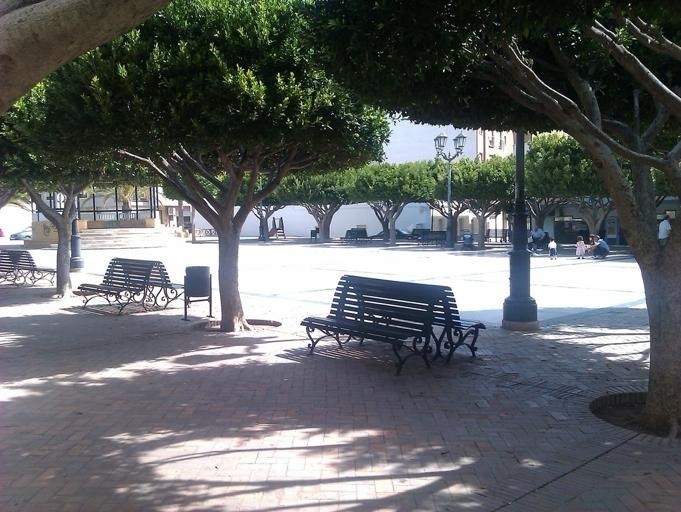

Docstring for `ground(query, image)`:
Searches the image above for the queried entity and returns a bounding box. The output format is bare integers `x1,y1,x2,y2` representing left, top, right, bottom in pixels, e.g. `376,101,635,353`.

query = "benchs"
77,257,184,316
299,275,487,377
411,228,447,248
0,249,58,287
340,228,373,244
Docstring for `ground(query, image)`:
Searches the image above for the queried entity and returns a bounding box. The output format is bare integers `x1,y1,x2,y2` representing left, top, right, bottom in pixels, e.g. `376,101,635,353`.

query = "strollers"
462,231,473,250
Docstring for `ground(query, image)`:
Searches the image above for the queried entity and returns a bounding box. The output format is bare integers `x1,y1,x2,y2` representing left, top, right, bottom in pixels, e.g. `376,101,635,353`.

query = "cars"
368,227,420,239
9,226,30,242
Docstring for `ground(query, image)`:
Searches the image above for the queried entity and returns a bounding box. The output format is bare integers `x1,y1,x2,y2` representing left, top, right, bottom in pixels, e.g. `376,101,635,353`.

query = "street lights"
434,129,468,247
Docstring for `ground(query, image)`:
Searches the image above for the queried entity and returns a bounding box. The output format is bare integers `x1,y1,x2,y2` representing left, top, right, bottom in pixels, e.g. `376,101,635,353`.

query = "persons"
588,236,610,259
548,238,558,261
575,236,586,259
659,214,672,249
530,225,546,250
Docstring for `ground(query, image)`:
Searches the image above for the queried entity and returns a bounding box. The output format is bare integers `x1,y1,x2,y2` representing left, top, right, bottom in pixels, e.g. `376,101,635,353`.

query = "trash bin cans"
181,266,214,320
311,230,317,240
463,233,473,245
